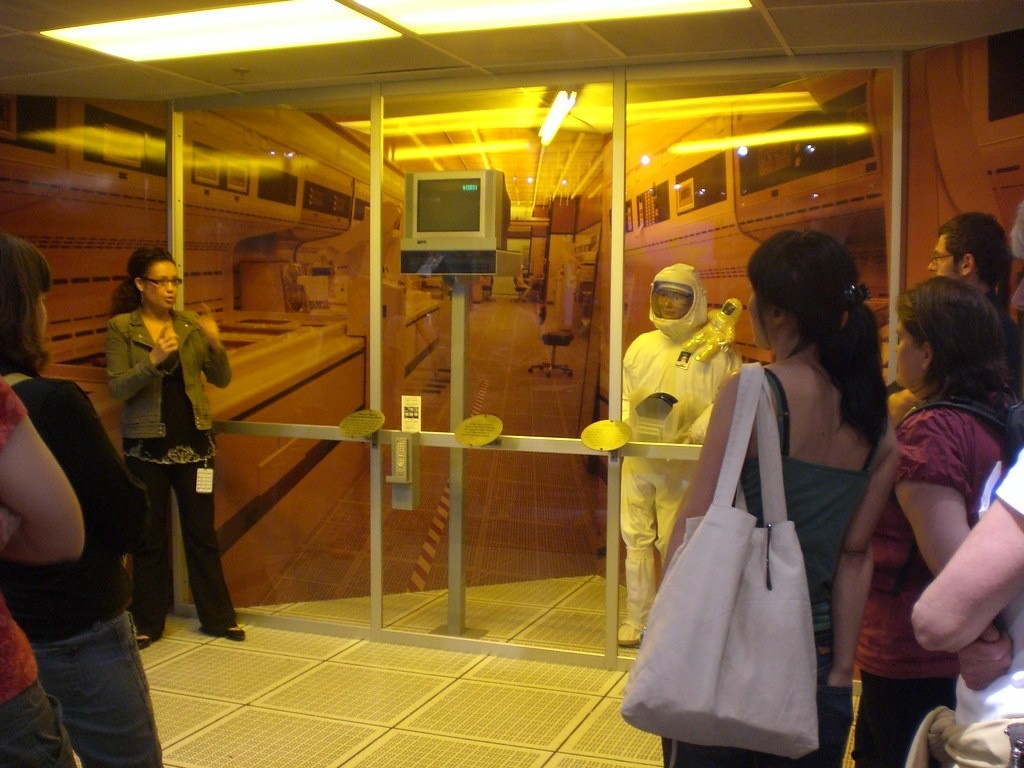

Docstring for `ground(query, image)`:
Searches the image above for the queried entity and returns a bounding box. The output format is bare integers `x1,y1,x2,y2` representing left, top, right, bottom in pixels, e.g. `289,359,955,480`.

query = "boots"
618,546,656,646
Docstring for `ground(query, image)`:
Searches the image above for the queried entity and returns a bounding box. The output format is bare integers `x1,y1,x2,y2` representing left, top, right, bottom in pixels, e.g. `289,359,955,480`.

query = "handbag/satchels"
621,359,820,758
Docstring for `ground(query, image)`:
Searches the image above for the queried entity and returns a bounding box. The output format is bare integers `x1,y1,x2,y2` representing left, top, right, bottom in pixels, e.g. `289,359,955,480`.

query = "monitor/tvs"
399,168,511,251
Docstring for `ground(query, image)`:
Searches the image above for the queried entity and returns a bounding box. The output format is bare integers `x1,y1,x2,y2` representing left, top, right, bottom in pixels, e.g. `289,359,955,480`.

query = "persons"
683,298,743,363
661,228,896,768
912,201,1024,768
0,234,162,768
928,212,1020,389
106,245,245,648
0,377,86,767
514,265,531,301
617,264,744,645
851,278,1007,767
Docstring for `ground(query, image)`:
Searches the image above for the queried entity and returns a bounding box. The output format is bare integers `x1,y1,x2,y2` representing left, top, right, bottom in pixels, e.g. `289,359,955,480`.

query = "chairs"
529,301,574,378
482,276,496,302
510,276,527,303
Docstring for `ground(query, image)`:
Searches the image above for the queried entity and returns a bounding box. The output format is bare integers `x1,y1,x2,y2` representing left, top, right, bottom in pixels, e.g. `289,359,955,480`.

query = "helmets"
648,264,709,338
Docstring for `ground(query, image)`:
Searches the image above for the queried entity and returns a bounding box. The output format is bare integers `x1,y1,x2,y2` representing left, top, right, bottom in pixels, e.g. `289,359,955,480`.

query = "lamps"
537,88,580,147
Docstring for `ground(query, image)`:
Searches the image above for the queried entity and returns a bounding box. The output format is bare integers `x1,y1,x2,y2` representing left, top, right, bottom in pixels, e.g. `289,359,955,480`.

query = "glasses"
929,253,954,265
141,275,182,290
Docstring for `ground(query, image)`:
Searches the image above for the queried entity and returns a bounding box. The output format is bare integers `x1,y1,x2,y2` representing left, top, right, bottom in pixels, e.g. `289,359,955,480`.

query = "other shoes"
199,624,245,641
135,632,163,650
